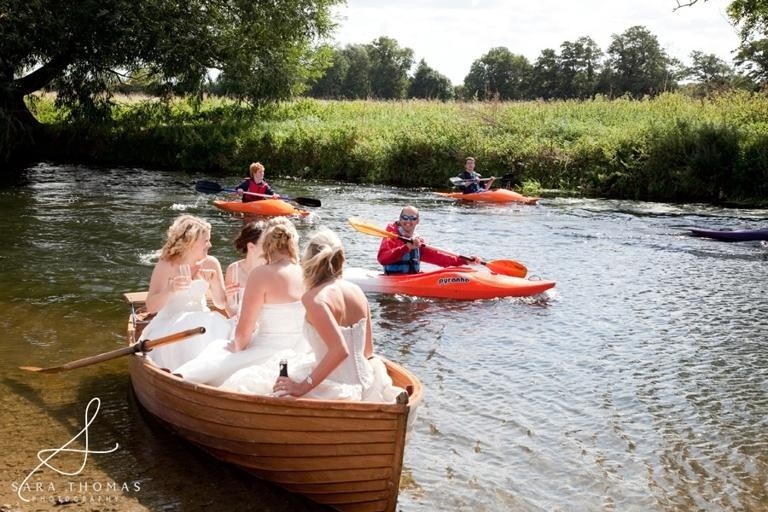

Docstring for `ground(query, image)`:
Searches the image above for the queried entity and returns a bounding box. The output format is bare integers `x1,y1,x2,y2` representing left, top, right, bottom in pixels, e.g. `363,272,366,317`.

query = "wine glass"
179,265,198,308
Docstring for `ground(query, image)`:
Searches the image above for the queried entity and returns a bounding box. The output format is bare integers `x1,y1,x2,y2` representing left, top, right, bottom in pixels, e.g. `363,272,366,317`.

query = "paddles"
348,217,527,278
449,173,513,186
18,327,206,372
195,180,321,208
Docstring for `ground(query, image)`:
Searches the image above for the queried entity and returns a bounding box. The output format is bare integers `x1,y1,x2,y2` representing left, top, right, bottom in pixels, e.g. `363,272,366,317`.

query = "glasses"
401,215,418,220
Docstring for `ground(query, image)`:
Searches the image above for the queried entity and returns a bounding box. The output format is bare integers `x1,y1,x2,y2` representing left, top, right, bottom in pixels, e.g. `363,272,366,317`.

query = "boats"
124,289,420,510
447,188,538,206
344,258,558,302
212,199,315,219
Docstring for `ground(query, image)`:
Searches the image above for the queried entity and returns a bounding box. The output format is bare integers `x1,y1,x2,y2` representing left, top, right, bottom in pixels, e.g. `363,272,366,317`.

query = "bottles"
274,359,291,398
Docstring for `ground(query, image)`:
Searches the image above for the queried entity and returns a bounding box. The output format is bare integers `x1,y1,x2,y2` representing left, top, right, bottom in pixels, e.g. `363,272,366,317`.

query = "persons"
135,162,405,404
377,206,482,275
453,157,496,193
